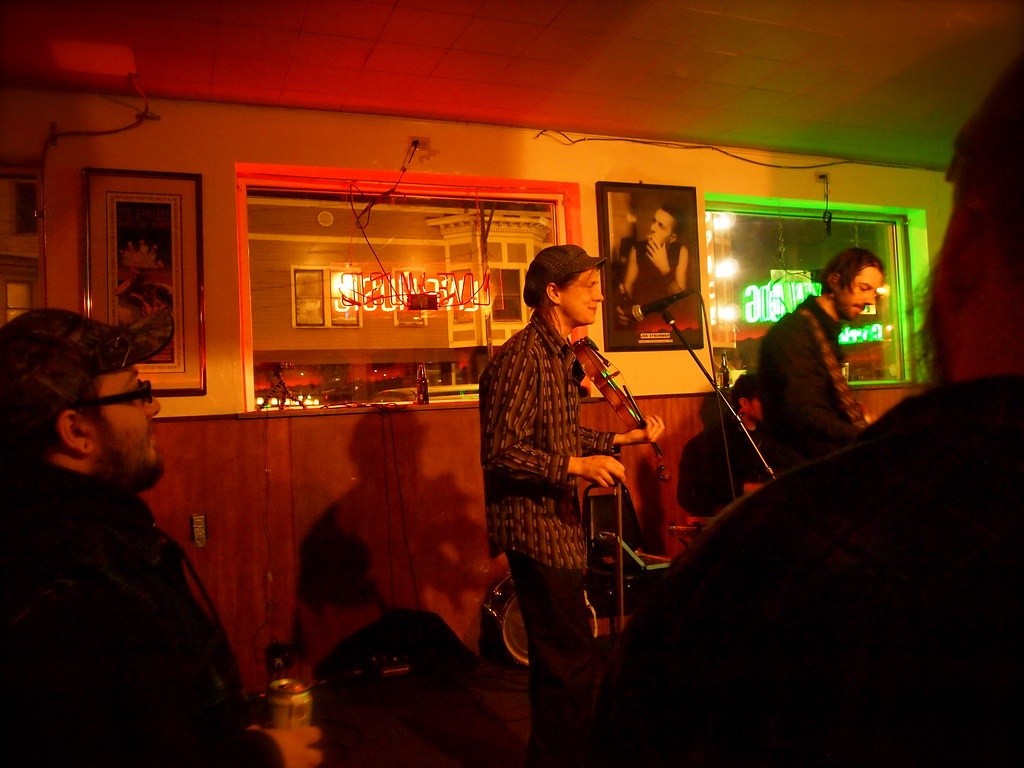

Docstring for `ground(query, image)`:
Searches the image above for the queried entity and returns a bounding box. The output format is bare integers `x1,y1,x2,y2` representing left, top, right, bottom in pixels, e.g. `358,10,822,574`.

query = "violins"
570,336,674,484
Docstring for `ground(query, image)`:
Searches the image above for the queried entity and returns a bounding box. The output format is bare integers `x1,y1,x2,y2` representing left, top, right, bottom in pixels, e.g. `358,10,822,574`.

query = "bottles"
617,282,638,327
415,362,430,405
717,353,729,389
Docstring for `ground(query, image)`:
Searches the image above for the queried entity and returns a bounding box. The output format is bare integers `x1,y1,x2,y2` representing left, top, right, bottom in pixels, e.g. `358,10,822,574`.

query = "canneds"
262,678,312,727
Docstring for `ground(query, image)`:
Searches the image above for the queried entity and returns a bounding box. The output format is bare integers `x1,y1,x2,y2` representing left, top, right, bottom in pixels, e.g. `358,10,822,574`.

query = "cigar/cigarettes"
645,234,653,238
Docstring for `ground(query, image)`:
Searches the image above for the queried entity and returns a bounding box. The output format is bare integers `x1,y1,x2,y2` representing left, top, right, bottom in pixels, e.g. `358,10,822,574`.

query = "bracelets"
663,270,675,283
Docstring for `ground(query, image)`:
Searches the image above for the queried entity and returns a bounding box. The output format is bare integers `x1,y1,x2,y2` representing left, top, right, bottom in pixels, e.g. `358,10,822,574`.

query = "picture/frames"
80,166,209,398
392,266,428,328
290,266,330,330
596,180,706,353
329,267,365,330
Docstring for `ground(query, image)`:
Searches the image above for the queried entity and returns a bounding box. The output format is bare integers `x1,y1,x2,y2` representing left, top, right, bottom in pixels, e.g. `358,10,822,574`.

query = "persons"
584,58,1024,768
614,205,689,328
477,244,666,768
0,309,325,768
712,374,791,514
759,249,887,468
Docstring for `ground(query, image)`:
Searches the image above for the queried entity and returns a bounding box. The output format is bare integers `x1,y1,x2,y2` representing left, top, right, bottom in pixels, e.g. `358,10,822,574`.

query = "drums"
485,566,598,670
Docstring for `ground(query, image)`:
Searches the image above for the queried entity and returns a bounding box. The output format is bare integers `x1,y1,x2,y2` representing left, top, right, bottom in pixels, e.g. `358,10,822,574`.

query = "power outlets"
815,171,830,184
409,135,432,152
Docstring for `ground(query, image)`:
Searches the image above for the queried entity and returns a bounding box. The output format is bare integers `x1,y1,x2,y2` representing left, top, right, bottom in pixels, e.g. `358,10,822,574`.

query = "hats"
0,308,176,438
523,244,608,307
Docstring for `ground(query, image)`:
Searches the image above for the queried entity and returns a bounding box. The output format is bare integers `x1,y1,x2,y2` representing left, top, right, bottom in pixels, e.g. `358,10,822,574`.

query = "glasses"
66,378,152,409
